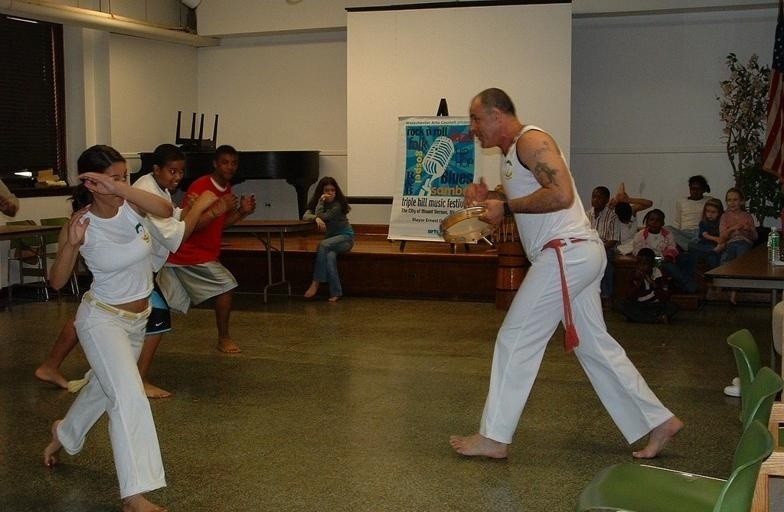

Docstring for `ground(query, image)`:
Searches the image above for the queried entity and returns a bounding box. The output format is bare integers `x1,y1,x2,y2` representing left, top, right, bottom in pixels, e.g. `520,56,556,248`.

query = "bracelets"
238,207,243,217
503,203,511,218
210,207,214,215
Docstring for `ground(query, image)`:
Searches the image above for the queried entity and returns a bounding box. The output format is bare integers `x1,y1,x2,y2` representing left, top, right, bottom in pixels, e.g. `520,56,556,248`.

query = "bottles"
768,226,780,264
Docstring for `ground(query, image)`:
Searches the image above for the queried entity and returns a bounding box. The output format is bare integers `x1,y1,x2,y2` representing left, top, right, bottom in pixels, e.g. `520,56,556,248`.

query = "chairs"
726,299,784,512
577,420,777,511
0,217,82,302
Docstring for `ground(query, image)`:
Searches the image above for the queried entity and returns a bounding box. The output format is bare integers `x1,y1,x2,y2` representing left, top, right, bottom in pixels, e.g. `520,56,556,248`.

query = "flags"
761,0,783,188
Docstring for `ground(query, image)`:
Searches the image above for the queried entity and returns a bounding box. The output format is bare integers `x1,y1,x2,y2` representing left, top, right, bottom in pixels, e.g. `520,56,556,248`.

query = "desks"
702,238,784,398
222,217,314,304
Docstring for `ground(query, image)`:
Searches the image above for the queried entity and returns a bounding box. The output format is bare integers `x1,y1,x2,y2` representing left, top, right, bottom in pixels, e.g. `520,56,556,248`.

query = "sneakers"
724,376,741,396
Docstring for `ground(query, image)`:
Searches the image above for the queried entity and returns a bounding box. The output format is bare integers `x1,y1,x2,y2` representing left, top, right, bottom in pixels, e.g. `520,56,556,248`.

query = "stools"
172,109,219,151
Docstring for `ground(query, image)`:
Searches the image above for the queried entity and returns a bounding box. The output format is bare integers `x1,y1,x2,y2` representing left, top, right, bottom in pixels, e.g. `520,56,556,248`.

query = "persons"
584,174,758,323
303,176,354,304
0,179,19,217
34,143,218,399
450,88,683,459
158,144,255,352
44,144,174,511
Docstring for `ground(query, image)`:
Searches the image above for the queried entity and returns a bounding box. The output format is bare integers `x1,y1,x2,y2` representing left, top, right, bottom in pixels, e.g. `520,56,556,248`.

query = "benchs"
600,254,672,312
